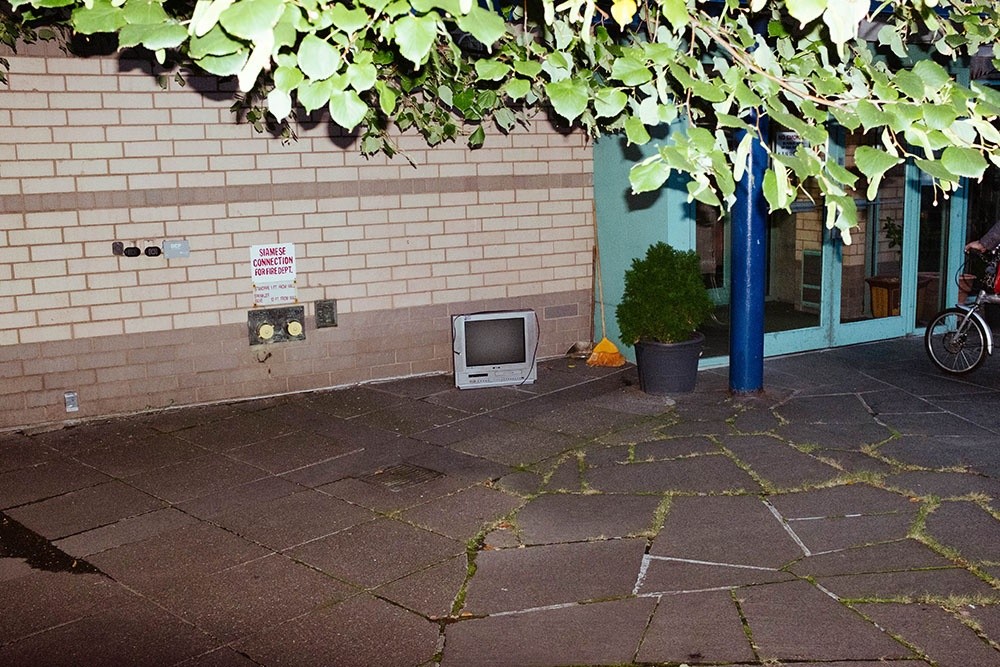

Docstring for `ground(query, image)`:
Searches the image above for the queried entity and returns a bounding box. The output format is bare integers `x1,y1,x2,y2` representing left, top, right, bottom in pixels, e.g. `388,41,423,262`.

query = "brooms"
584,199,626,367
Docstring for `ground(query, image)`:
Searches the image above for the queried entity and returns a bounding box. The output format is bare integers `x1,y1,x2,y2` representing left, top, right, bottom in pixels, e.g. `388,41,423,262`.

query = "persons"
965,221,1000,254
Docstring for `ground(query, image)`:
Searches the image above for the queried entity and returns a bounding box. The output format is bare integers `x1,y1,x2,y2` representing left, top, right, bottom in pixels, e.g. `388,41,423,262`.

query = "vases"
922,271,976,322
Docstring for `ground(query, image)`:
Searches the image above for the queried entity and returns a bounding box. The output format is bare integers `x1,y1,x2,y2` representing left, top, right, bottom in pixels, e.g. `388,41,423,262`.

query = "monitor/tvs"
451,309,537,390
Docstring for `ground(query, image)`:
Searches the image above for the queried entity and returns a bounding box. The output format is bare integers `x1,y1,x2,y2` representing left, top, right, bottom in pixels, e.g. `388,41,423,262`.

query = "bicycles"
924,240,1000,376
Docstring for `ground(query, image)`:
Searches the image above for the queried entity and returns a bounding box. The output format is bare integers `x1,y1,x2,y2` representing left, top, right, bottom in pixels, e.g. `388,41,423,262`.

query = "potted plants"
614,240,717,395
864,216,931,327
